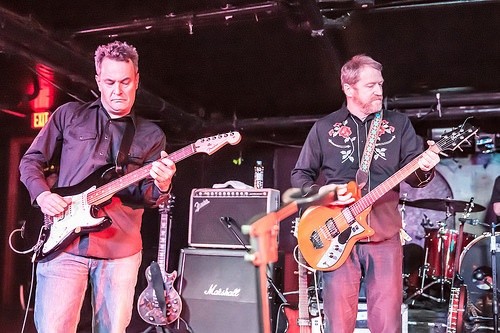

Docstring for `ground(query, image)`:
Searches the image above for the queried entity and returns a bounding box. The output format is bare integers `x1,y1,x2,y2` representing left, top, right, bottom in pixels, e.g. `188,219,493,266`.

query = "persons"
19,42,176,333
291,55,440,333
486,175,500,231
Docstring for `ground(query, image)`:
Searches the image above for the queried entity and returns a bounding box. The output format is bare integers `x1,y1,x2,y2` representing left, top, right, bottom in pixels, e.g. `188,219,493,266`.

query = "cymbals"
399,197,416,207
415,198,486,213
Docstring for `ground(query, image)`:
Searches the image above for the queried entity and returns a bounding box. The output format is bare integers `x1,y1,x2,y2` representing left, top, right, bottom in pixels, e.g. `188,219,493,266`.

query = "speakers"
168,249,279,333
189,187,281,250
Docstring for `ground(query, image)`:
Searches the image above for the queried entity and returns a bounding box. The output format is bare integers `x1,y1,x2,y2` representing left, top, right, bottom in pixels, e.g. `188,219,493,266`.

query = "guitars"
274,217,323,333
136,194,184,326
296,115,479,272
23,130,243,263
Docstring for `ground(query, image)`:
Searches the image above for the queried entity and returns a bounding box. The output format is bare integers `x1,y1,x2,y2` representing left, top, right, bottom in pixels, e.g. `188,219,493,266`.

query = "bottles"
253,161,264,189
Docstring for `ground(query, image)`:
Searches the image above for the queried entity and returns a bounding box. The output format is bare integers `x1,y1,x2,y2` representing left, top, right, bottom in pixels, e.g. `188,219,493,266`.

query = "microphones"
221,216,233,222
436,93,442,117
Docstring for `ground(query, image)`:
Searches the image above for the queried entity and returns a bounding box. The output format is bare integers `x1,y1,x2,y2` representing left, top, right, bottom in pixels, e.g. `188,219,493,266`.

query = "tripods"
403,204,453,307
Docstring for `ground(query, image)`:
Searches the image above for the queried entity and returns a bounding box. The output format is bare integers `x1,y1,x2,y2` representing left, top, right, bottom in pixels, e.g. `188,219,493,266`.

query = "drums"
424,227,477,282
402,243,428,305
459,231,500,318
